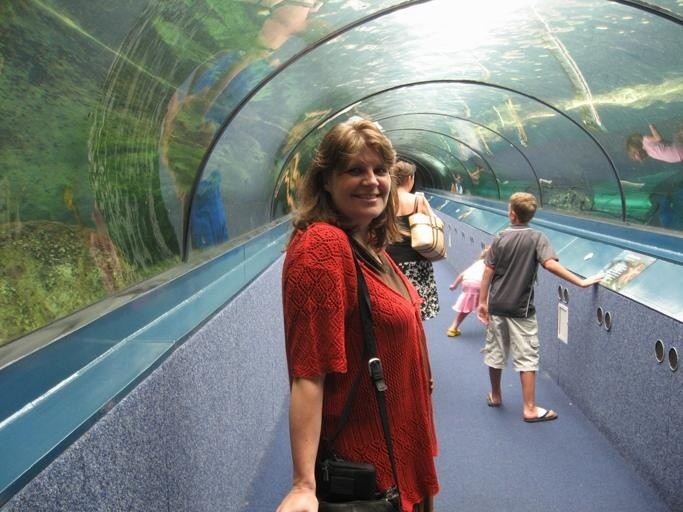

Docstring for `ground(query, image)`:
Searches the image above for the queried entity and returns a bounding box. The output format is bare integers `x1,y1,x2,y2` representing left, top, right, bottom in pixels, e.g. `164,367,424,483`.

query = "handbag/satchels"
409,213,448,262
314,457,402,512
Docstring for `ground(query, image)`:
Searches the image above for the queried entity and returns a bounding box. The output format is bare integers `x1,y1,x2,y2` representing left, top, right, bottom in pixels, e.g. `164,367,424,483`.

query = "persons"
475,191,607,423
273,114,441,512
389,160,440,321
626,124,683,164
446,243,493,337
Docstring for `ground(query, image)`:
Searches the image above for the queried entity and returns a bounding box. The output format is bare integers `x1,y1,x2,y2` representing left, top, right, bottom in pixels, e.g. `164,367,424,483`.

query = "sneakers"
447,329,461,337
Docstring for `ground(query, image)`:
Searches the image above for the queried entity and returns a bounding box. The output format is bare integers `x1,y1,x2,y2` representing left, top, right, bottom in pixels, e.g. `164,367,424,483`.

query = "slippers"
487,393,497,406
524,407,557,422
